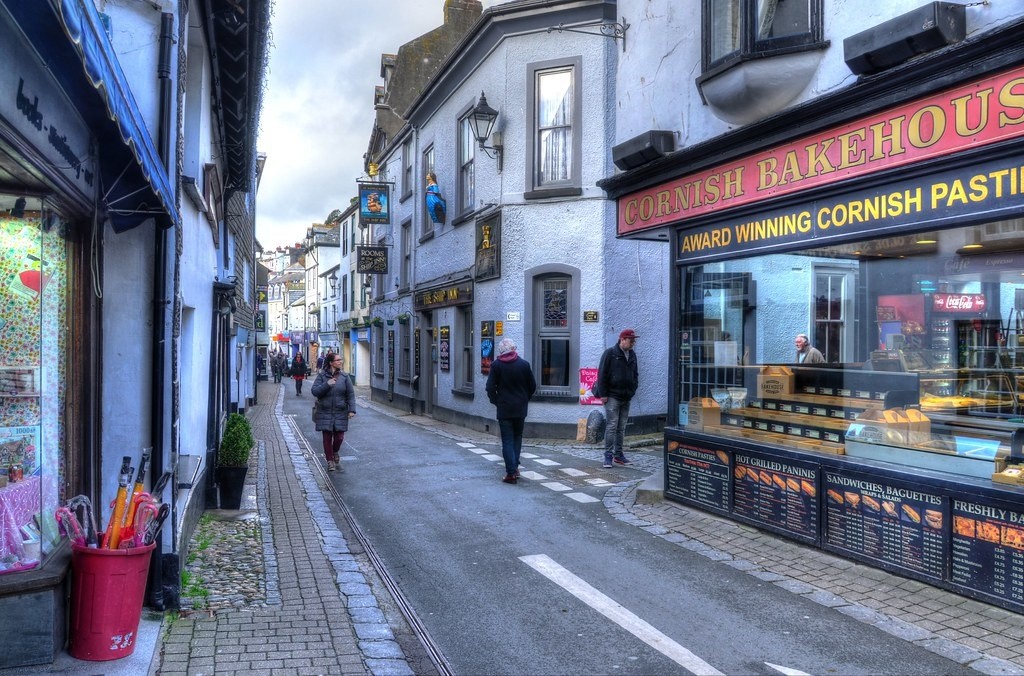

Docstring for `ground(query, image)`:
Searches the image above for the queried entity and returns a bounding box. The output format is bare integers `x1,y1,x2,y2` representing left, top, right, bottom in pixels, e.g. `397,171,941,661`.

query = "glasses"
297,354,300,355
333,359,342,362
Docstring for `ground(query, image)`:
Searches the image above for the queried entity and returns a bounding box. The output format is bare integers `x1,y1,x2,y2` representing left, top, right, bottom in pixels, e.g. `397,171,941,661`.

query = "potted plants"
373,316,382,327
399,314,407,324
215,412,256,509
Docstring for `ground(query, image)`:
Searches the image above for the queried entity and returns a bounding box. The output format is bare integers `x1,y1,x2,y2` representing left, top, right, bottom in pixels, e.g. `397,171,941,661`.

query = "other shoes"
327,452,339,471
503,469,521,484
296,391,302,396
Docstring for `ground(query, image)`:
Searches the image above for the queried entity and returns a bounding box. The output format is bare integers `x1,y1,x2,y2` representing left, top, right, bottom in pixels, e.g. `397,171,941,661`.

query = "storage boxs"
0,366,40,398
0,425,40,477
688,366,930,455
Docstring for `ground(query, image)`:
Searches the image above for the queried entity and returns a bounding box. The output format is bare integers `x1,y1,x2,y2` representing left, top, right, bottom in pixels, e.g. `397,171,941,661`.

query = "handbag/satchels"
312,401,318,423
592,381,600,398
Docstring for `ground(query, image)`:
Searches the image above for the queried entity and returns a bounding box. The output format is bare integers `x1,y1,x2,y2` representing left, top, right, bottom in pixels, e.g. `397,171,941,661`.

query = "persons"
311,352,356,471
425,173,446,223
268,351,288,383
597,329,641,469
793,334,826,364
485,338,537,485
315,349,333,374
290,351,308,396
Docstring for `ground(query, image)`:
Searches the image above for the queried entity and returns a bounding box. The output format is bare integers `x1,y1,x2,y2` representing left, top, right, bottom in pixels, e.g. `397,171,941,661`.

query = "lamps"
703,289,713,297
10,195,26,218
36,208,58,235
328,270,341,289
403,310,419,319
467,90,503,176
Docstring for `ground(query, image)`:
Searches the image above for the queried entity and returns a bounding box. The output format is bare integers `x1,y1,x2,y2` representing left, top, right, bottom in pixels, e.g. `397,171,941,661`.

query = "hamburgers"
734,466,746,479
924,510,942,530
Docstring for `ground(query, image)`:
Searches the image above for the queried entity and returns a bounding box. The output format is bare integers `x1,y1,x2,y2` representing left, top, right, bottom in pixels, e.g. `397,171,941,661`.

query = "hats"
620,329,640,339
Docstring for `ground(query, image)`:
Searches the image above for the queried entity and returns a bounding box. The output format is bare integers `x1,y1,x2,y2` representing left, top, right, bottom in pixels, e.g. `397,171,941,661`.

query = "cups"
23,539,41,563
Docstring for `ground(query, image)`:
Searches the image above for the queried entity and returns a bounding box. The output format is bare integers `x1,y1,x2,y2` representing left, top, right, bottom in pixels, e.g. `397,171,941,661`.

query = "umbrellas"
55,454,170,551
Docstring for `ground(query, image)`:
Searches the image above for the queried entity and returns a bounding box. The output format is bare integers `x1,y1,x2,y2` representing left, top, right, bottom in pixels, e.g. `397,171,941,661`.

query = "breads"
960,401,977,406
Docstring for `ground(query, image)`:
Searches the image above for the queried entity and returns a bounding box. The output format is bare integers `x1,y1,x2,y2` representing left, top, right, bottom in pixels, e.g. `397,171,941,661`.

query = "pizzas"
957,517,1024,550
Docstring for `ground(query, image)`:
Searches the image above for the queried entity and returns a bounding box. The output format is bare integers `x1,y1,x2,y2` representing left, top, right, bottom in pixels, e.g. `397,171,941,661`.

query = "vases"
387,320,394,325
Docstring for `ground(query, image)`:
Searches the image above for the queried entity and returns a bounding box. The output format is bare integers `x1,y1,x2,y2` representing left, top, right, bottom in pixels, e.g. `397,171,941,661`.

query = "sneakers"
614,456,633,466
603,456,613,468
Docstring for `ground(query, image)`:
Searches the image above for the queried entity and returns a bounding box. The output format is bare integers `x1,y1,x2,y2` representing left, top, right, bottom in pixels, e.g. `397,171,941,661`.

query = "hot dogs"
746,466,921,524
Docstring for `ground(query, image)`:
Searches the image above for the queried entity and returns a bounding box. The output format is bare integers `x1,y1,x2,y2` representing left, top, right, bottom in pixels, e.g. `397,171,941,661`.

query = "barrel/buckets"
67,533,157,660
